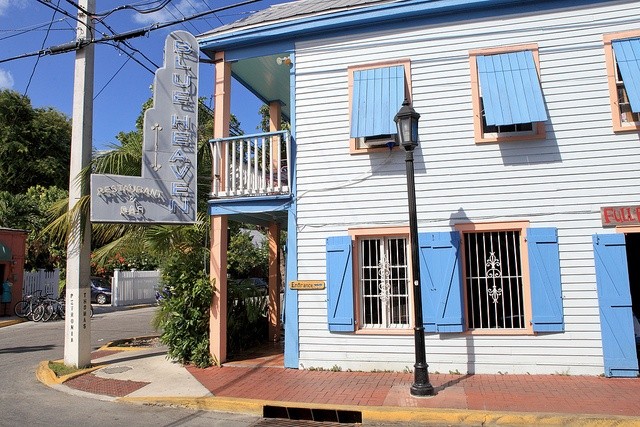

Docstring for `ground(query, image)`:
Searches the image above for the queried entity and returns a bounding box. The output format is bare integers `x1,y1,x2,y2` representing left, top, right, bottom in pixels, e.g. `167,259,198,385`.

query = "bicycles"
13,287,65,323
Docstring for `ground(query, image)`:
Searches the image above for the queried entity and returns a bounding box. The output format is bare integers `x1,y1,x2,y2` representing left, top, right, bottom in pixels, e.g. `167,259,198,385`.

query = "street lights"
393,97,435,395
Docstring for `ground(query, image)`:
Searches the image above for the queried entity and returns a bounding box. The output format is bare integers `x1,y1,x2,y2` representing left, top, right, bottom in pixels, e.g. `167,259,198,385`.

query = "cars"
91,276,111,303
